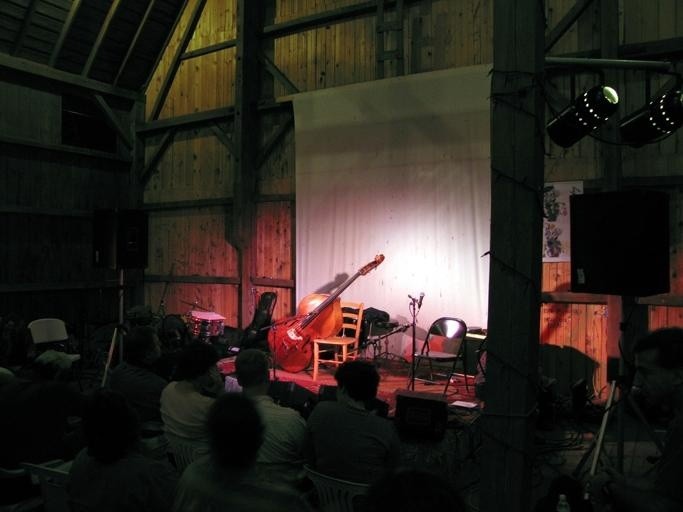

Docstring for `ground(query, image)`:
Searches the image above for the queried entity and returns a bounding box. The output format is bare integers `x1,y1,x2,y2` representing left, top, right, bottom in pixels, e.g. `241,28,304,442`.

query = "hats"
177,340,226,377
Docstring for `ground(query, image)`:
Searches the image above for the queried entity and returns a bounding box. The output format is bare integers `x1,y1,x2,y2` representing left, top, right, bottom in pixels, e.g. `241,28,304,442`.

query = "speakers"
570,189,670,297
91,209,148,270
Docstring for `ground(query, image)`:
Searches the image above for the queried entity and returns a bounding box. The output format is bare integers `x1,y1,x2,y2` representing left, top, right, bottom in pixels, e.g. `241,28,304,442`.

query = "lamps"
545,71,683,149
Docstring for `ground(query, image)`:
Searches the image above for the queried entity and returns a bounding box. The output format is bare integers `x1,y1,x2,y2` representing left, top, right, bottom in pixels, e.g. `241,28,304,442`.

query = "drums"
184,311,226,343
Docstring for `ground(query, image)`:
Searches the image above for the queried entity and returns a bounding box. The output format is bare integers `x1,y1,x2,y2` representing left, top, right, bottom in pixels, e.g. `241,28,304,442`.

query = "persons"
631,326,682,510
0,306,412,512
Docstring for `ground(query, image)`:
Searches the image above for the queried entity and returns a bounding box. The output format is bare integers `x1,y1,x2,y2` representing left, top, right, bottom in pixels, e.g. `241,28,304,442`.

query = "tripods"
101,270,126,388
573,295,663,485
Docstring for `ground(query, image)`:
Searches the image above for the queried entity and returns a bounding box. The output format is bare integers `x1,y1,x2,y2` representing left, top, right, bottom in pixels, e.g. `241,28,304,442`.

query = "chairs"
1,458,80,512
305,463,370,512
310,300,363,379
26,318,86,392
158,407,209,483
403,316,472,398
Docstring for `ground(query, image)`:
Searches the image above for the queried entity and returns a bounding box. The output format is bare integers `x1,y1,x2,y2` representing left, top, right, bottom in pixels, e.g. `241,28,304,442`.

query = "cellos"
265,253,388,372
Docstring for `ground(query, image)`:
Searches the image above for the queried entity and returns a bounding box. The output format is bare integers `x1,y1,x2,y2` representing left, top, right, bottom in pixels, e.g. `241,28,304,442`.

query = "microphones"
418,292,425,308
